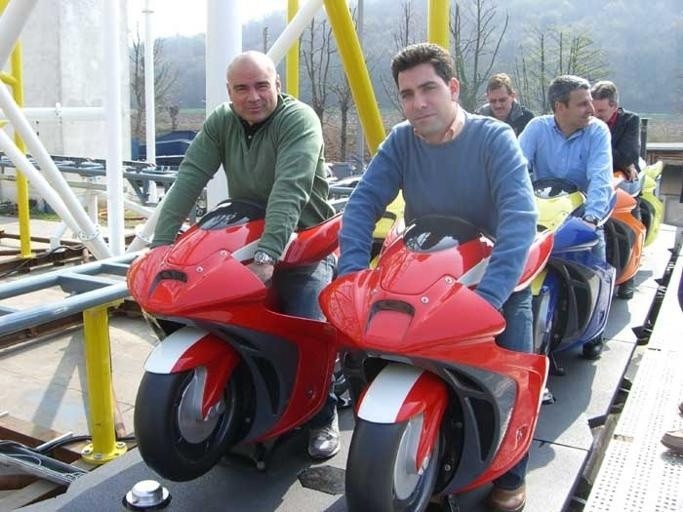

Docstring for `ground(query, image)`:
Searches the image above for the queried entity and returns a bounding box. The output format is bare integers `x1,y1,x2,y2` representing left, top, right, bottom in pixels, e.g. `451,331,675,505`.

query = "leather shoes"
490,449,530,512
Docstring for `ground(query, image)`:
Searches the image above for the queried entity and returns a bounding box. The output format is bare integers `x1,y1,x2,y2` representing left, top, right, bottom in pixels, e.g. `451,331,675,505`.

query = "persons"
516,73,615,358
661,268,683,454
589,78,641,298
149,50,344,462
335,40,540,512
472,71,536,140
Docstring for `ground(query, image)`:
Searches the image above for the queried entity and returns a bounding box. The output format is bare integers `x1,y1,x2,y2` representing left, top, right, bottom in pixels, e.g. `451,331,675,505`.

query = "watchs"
582,214,600,228
252,251,275,266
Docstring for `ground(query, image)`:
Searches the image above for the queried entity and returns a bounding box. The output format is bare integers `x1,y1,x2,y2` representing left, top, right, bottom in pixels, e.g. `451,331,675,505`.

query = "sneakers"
582,333,602,358
618,277,634,301
308,407,339,461
